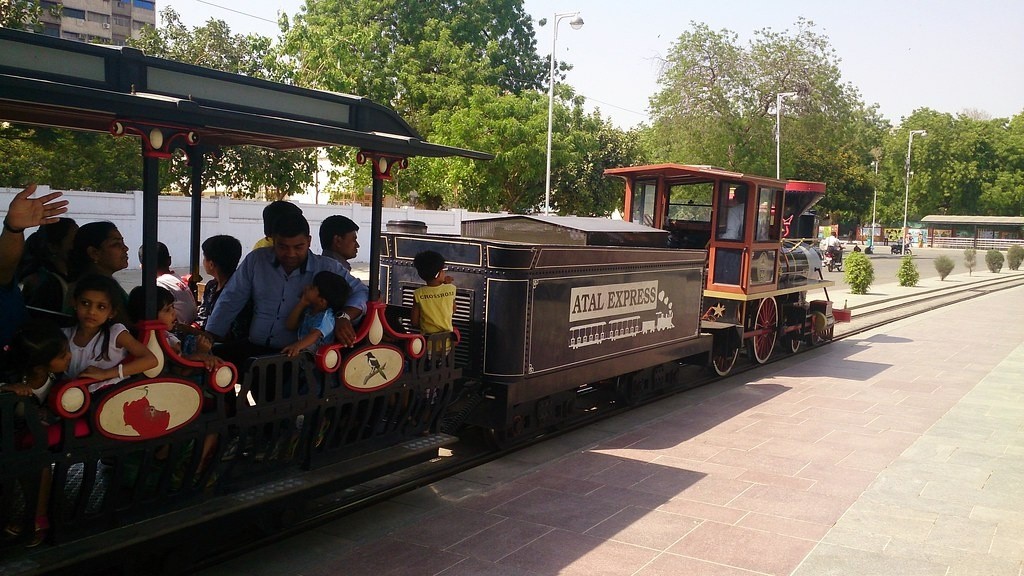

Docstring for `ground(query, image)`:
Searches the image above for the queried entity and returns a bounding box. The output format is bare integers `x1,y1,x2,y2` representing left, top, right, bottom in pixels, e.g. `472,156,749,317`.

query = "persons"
884,230,890,246
0,183,458,540
705,184,746,266
918,234,923,248
827,232,845,266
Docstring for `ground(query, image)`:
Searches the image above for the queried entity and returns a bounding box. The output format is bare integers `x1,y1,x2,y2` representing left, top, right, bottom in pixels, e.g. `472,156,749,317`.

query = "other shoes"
176,418,332,491
24,522,54,547
3,517,28,537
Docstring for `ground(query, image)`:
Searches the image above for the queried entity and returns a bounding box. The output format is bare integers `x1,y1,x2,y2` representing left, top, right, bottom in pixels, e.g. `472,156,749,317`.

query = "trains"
0,22,852,576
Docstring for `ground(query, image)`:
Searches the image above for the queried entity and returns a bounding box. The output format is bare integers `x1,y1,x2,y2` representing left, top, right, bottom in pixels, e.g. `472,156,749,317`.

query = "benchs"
0,304,457,551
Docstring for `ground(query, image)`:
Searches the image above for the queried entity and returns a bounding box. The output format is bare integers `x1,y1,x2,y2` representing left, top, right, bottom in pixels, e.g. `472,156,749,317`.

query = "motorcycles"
825,247,846,272
891,241,912,255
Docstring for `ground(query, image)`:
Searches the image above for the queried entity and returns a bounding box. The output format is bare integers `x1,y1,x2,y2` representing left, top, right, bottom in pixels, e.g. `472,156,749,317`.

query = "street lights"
775,91,812,181
901,129,928,255
870,160,879,251
544,10,584,216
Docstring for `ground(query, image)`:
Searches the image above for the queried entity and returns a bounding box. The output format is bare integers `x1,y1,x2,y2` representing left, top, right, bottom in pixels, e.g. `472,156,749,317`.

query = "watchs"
335,310,351,321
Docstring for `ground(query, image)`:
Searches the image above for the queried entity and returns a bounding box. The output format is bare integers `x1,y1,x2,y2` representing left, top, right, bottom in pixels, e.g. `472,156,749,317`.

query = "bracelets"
192,330,195,333
118,363,124,378
4,216,25,233
